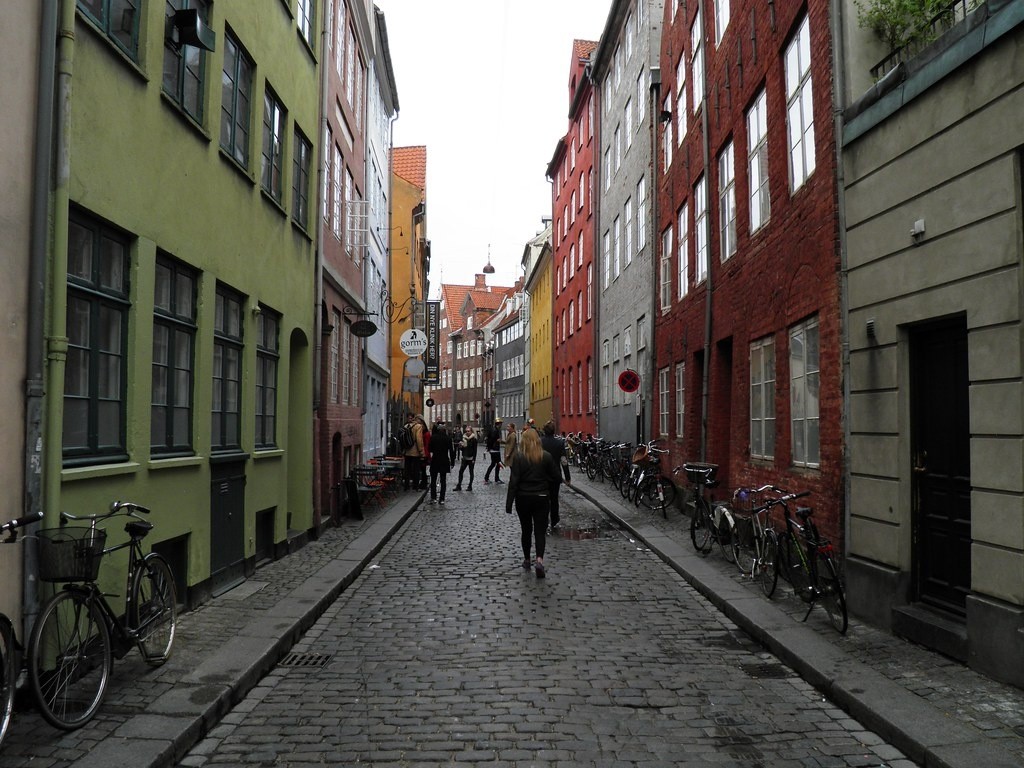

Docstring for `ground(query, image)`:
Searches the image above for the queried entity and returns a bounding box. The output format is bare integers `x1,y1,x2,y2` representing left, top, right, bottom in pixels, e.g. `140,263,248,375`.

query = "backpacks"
397,423,417,455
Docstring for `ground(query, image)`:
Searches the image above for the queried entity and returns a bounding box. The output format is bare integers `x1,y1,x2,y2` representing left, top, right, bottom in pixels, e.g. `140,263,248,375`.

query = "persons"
541,422,571,528
505,429,561,578
504,423,518,467
453,425,463,460
405,413,442,488
404,414,425,492
484,419,505,483
453,426,477,491
428,424,456,505
562,431,604,461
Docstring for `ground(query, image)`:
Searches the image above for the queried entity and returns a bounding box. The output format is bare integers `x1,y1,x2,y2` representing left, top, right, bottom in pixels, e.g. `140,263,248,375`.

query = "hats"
495,418,503,423
415,414,424,420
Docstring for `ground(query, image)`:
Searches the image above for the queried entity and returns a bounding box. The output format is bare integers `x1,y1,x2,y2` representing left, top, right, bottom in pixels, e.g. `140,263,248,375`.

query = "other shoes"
466,485,472,491
522,560,531,570
495,480,504,484
429,499,437,503
551,517,560,527
534,562,545,578
484,478,492,483
438,500,444,504
453,484,462,491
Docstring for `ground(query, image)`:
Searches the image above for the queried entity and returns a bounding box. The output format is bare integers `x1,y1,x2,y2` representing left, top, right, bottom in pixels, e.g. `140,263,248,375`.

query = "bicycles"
751,488,848,635
556,431,678,519
731,485,786,600
672,461,741,565
0,511,46,754
29,500,178,732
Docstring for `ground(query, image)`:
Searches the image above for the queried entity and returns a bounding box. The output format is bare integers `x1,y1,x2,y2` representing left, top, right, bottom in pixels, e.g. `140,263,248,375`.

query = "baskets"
32,526,107,584
632,446,651,468
686,461,719,486
